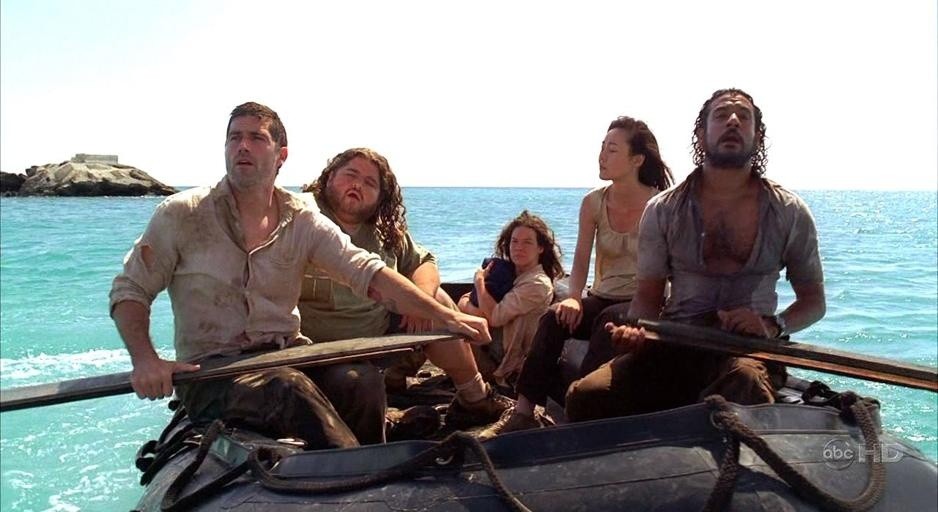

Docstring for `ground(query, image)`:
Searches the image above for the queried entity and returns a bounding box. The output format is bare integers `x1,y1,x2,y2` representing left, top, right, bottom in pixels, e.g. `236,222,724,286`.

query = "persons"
295,146,519,441
564,86,827,418
476,114,676,439
459,209,565,397
105,101,494,448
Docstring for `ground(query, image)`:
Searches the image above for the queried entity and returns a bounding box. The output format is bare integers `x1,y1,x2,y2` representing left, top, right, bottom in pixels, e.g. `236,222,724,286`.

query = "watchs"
762,312,788,339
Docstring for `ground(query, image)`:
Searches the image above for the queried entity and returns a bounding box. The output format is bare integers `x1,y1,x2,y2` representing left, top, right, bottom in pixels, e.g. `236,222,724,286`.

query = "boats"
134,282,938,511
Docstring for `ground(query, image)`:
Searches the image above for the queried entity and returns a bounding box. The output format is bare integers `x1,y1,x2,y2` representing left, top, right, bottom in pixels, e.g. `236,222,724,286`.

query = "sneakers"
446,382,517,430
477,407,541,440
